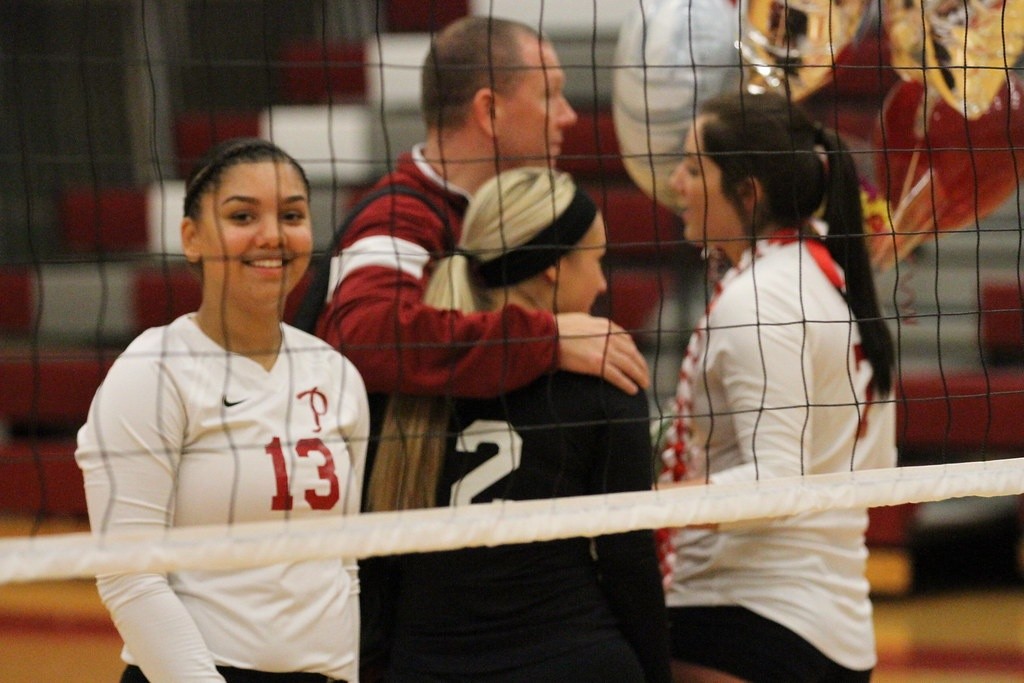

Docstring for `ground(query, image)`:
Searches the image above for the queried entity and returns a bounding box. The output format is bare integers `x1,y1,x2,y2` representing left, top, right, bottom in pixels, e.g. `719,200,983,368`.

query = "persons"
75,139,369,683
646,93,894,683
289,17,669,683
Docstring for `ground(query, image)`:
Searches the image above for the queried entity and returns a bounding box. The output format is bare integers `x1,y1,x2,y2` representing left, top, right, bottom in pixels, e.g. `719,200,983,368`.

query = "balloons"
612,0,1024,273
